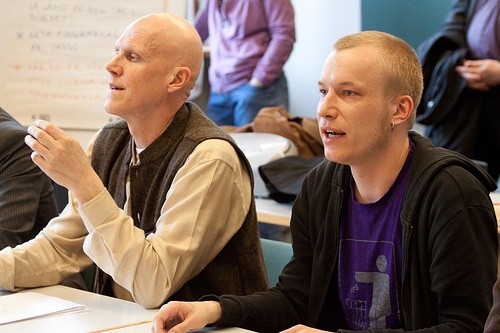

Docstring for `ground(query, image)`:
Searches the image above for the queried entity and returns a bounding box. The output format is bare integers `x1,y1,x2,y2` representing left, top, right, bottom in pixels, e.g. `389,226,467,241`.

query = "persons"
485,279,500,333
191,0,296,130
416,0,500,182
0,107,88,293
150,30,498,333
0,13,271,309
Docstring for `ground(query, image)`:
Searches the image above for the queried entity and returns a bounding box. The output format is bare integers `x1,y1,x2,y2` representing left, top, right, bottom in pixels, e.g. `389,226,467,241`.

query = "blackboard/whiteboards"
0,1,187,132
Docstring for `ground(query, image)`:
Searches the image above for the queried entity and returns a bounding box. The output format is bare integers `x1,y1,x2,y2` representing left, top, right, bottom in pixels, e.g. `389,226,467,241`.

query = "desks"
252,197,294,244
0,272,276,333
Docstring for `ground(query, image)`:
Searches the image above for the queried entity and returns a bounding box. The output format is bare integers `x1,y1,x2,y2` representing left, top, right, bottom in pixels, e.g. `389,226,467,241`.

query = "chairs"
258,238,295,287
